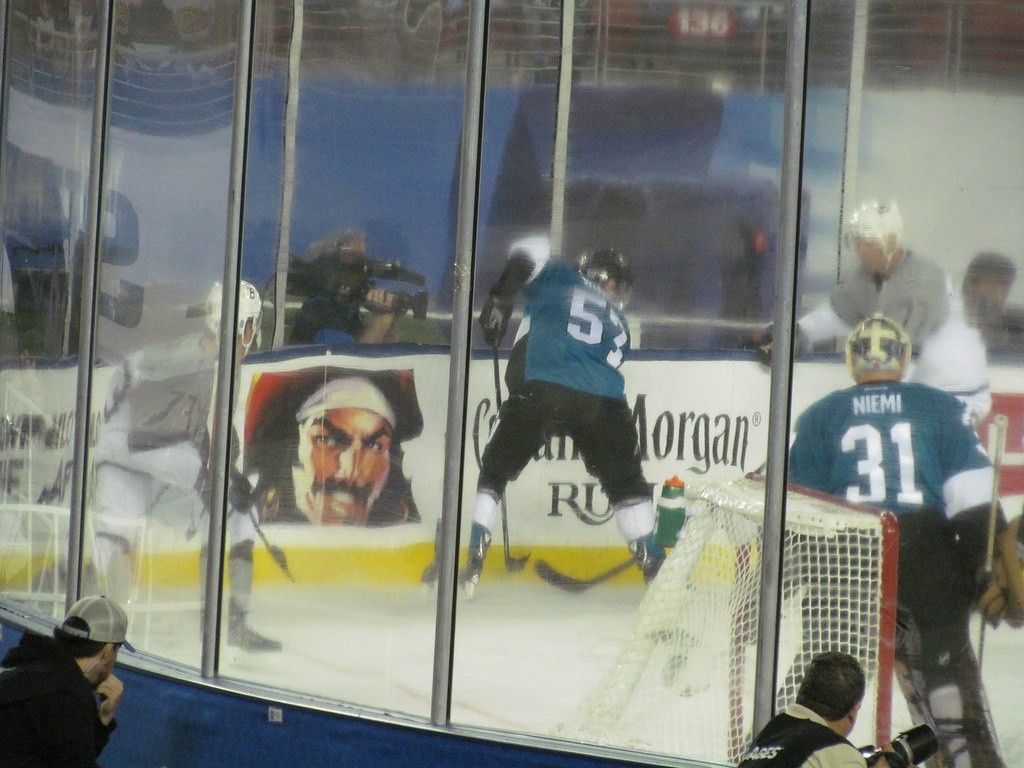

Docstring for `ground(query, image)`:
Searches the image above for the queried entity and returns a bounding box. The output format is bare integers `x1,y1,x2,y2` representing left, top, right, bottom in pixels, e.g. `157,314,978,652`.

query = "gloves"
974,553,1010,629
479,292,513,351
227,468,258,515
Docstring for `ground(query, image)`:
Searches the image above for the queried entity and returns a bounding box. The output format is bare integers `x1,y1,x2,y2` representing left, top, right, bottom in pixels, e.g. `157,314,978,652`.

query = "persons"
0,593,137,763
758,202,947,368
908,252,1015,428
104,279,285,654
737,653,918,768
292,228,396,345
776,312,1008,768
423,236,667,586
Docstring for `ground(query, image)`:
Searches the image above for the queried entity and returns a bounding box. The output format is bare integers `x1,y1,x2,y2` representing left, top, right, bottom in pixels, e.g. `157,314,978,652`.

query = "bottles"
651,477,685,546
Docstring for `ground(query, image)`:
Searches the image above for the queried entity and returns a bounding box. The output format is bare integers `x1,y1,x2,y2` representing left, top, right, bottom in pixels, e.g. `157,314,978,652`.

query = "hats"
60,595,136,653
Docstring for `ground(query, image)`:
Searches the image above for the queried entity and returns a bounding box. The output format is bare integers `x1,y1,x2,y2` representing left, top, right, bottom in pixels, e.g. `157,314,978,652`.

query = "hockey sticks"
487,319,533,573
975,412,1010,675
529,458,767,596
244,504,299,587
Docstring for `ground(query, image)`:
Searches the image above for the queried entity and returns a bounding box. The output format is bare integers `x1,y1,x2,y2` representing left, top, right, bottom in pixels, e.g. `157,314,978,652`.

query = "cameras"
858,723,939,768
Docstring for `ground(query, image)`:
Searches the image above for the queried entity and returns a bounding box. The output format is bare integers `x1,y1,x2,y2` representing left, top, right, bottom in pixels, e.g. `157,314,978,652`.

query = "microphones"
366,265,426,286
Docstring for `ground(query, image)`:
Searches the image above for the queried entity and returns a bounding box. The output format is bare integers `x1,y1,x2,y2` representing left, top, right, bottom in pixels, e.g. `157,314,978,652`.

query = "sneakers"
418,520,492,599
626,531,699,613
224,596,285,653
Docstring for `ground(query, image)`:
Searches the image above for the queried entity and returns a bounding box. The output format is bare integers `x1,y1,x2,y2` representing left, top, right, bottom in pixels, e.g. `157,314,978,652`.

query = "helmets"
578,247,632,292
849,200,903,239
204,280,264,335
845,312,912,377
